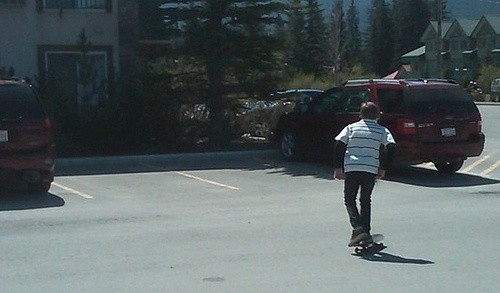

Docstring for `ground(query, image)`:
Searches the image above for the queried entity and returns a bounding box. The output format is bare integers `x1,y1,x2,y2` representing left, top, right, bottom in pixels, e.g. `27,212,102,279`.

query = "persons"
333,101,396,246
471,80,477,90
468,80,473,87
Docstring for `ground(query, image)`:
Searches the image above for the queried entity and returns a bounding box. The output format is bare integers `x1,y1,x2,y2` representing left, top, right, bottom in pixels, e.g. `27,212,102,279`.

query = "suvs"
275,82,485,179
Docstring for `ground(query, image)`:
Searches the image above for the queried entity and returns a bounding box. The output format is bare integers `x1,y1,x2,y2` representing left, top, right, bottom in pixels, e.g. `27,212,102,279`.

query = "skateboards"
348,232,384,250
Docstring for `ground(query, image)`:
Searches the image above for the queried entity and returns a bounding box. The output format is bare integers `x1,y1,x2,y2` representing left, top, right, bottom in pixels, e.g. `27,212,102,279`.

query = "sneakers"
360,235,373,244
349,227,370,247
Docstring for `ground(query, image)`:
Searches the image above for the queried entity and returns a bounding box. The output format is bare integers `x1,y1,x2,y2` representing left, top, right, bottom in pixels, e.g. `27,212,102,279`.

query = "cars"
0,74,58,198
249,90,323,137
